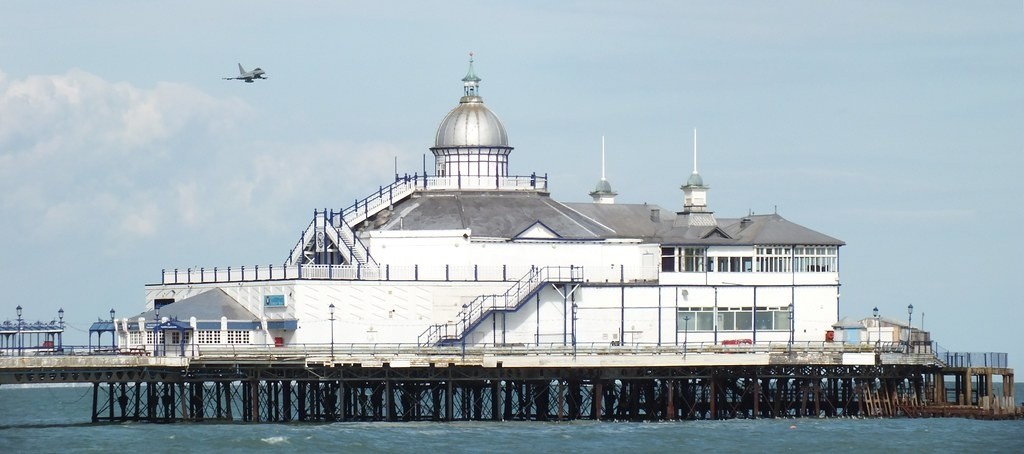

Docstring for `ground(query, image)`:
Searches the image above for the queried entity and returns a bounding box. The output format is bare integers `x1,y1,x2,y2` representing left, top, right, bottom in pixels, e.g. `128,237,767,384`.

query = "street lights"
154,305,161,355
906,304,914,352
328,302,336,361
109,308,116,352
872,306,879,317
57,308,65,350
15,305,24,355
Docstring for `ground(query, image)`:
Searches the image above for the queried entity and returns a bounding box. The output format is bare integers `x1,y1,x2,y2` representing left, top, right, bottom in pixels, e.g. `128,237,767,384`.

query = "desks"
117,351,151,356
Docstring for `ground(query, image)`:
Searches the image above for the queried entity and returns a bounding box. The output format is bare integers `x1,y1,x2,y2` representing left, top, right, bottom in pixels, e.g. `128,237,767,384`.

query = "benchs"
94,348,120,354
32,350,57,356
130,345,147,357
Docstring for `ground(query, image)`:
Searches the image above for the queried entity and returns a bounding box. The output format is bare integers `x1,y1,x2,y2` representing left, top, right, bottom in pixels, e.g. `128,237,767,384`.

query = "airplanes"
221,61,269,85
